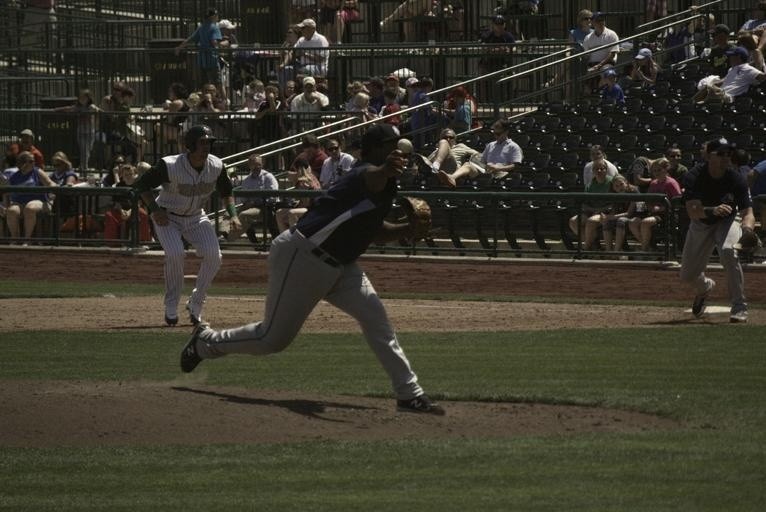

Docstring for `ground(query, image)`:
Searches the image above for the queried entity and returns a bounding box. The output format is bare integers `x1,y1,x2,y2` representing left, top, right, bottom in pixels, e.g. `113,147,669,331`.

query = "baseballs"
399,139,412,153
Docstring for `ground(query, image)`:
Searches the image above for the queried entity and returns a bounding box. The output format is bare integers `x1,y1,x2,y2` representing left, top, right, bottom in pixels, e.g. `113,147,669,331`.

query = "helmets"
184,125,218,155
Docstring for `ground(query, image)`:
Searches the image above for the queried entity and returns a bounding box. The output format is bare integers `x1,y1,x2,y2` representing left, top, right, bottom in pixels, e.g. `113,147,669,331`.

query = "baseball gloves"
400,195,431,246
733,227,762,254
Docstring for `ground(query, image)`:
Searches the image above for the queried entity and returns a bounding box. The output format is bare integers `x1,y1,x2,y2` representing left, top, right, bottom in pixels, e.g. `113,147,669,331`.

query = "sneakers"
437,170,457,190
688,280,715,319
180,323,211,372
163,306,181,325
730,308,750,323
394,396,446,417
185,304,204,326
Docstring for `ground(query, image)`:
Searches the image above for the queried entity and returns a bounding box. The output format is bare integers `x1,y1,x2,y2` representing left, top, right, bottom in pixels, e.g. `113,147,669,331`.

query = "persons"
48,151,85,239
745,159,766,232
364,77,386,116
681,5,699,60
704,24,737,79
379,87,401,126
480,16,516,46
218,18,238,49
450,87,471,134
583,145,618,187
276,158,322,234
384,74,408,106
439,119,524,191
7,151,57,248
133,124,242,327
319,140,355,190
276,18,330,99
398,77,419,106
239,154,279,251
690,46,766,111
629,157,681,262
569,159,613,260
280,24,301,62
602,70,625,107
666,149,686,182
55,88,104,170
601,174,641,261
579,12,620,95
291,134,328,180
350,92,372,116
408,77,433,153
97,75,329,165
173,7,230,105
179,124,445,416
6,128,45,171
410,129,478,178
679,137,755,323
103,165,153,241
736,3,766,47
631,47,665,89
102,155,128,187
540,10,594,88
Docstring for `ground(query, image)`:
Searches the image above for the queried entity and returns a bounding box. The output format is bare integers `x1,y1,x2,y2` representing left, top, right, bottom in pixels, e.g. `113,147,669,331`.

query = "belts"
159,206,194,218
290,222,354,270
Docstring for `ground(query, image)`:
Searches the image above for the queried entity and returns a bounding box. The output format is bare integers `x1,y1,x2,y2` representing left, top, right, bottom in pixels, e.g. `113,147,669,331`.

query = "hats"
706,23,732,39
20,129,33,140
634,47,652,61
219,20,236,31
603,70,618,78
725,45,750,63
301,76,317,88
405,76,422,88
384,73,399,83
362,124,413,151
396,138,416,155
590,10,607,22
297,19,316,31
492,14,505,29
706,138,737,154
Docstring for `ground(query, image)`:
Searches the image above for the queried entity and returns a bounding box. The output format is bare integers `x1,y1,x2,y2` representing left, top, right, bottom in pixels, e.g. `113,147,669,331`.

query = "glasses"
495,129,507,136
710,151,735,158
442,135,456,140
580,17,594,21
325,145,340,152
493,21,505,25
25,160,35,164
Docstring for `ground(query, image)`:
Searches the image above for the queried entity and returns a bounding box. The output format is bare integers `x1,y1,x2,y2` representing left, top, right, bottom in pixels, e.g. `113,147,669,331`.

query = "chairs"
424,58,766,256
